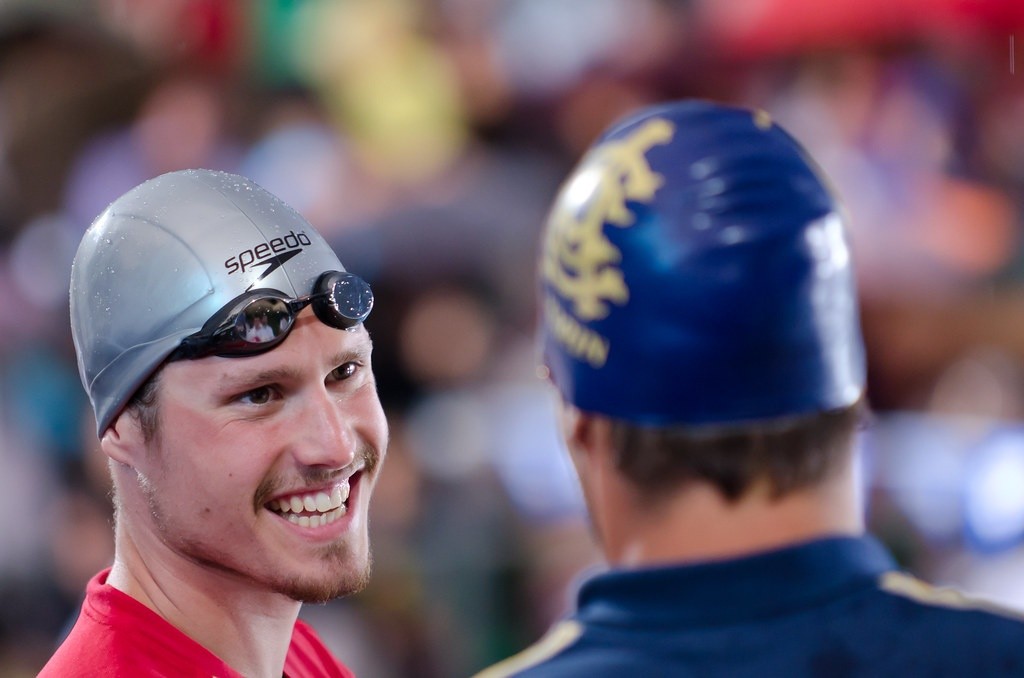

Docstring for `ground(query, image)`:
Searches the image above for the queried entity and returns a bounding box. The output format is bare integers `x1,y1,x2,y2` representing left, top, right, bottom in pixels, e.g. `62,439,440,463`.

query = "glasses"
176,271,374,360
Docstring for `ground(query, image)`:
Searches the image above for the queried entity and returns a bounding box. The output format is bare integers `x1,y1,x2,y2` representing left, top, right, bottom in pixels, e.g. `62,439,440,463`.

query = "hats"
70,168,350,432
533,92,874,422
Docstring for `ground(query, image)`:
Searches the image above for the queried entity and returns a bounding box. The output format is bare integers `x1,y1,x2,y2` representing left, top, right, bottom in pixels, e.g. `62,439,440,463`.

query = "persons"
3,3,1024,615
26,166,390,677
471,99,1021,677
